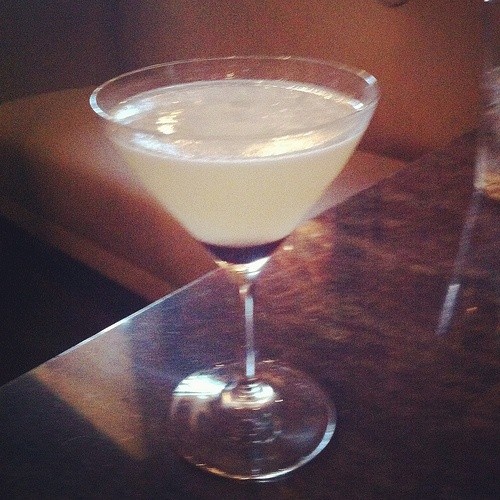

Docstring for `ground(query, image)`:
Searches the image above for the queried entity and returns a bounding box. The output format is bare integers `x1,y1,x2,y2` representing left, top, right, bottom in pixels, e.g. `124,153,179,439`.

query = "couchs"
1,0,500,304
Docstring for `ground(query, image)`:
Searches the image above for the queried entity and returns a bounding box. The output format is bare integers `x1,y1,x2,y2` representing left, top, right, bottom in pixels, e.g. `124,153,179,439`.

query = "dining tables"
1,125,500,500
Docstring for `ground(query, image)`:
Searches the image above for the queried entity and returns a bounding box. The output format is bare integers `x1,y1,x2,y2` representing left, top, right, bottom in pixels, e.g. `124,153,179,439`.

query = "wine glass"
89,56,383,484
